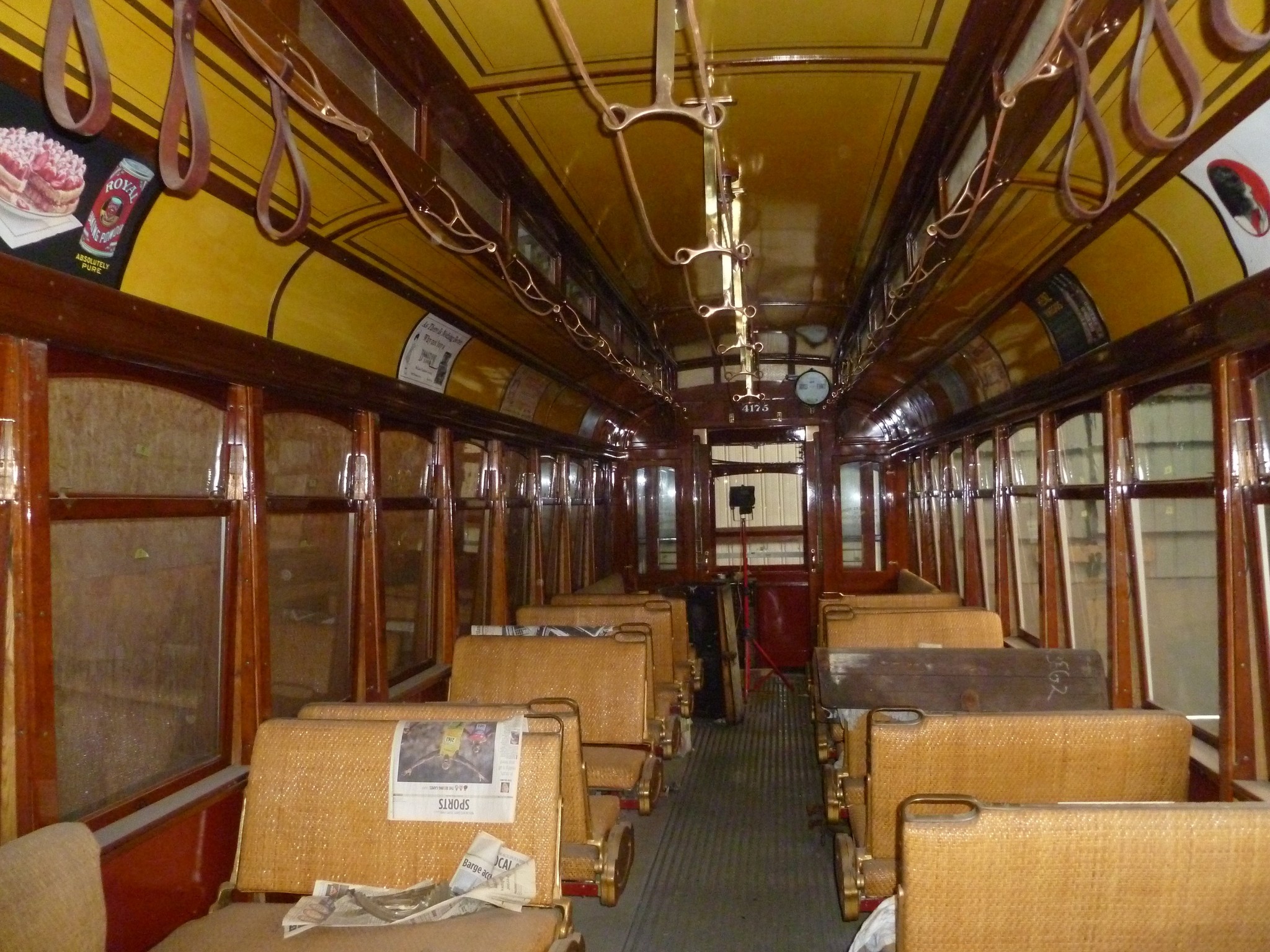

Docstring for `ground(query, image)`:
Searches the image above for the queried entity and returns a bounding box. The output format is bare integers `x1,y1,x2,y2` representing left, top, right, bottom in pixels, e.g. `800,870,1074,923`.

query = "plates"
0,197,73,220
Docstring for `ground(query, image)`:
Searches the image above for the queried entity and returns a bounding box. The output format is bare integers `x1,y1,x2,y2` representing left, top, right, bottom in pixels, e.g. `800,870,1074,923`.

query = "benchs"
0,821,109,952
804,569,1270,951
144,576,706,951
